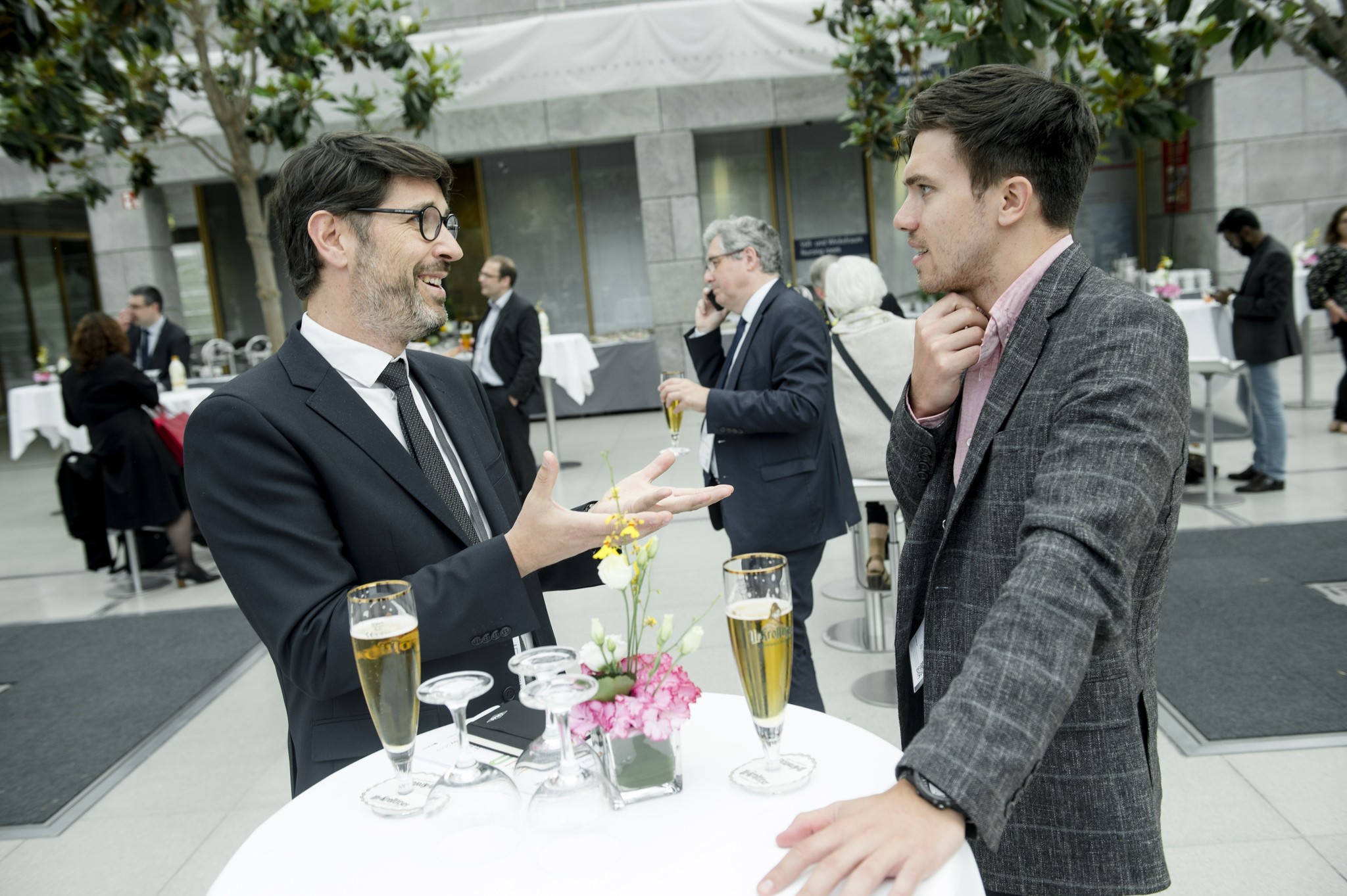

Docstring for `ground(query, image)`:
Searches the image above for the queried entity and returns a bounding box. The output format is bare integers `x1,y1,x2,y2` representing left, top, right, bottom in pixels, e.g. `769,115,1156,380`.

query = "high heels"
866,555,893,589
174,562,220,588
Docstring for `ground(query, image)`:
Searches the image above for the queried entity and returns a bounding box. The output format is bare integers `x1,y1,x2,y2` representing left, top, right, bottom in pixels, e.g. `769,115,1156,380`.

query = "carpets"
1151,516,1347,755
1,603,268,840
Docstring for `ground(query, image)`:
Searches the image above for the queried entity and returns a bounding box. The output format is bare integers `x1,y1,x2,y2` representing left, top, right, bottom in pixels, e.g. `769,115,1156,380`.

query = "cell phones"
707,289,724,311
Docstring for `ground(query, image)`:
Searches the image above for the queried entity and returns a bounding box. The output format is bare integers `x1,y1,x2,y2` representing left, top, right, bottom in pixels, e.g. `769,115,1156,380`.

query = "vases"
591,724,683,811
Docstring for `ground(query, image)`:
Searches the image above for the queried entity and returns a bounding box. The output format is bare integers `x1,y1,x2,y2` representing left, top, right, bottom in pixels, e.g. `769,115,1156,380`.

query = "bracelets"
897,768,968,816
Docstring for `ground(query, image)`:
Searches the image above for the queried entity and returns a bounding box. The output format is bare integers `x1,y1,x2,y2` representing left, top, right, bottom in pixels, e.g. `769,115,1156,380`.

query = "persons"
1307,206,1347,432
811,255,915,591
1209,209,1304,493
757,62,1191,896
471,257,542,501
658,214,863,714
63,285,222,589
183,131,732,801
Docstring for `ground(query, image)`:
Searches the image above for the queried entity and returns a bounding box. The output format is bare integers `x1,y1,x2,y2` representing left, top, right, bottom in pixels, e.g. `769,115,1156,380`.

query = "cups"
460,321,473,350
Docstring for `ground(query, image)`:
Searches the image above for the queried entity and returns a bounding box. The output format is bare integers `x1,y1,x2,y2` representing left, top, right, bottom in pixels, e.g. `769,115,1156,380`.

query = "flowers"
31,345,52,386
1153,254,1181,302
1299,227,1322,269
565,448,702,742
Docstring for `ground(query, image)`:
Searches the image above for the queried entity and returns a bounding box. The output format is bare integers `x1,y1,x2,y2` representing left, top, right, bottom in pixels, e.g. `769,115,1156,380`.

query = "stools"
1181,357,1248,510
821,474,901,709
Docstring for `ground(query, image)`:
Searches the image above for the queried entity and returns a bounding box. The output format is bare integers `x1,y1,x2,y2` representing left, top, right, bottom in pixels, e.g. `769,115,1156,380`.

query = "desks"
202,690,986,895
6,382,215,462
454,333,600,472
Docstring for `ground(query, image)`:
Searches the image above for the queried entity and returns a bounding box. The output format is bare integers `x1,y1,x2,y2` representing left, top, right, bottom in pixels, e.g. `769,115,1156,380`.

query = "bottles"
168,355,187,392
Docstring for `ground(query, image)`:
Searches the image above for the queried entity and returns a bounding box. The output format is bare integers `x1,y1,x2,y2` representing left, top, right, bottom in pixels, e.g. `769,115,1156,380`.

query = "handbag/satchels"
150,404,190,466
109,529,177,574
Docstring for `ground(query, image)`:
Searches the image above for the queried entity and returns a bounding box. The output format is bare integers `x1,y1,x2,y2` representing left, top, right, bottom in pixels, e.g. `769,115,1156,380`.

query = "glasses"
347,203,461,241
703,246,749,274
476,272,498,283
127,301,153,310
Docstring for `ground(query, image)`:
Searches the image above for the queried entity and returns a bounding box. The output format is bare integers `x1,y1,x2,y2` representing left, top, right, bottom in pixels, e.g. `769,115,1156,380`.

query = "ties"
698,316,749,390
471,307,496,374
378,358,484,548
138,327,152,370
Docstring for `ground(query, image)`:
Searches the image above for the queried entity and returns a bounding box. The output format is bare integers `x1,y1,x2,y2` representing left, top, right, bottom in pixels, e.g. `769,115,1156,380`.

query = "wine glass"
346,578,448,814
721,551,811,795
415,669,524,876
508,645,626,882
659,371,690,456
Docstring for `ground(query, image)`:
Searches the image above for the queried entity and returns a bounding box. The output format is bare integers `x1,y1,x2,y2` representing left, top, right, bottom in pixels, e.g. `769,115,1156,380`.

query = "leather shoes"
1228,465,1258,481
1235,474,1284,493
